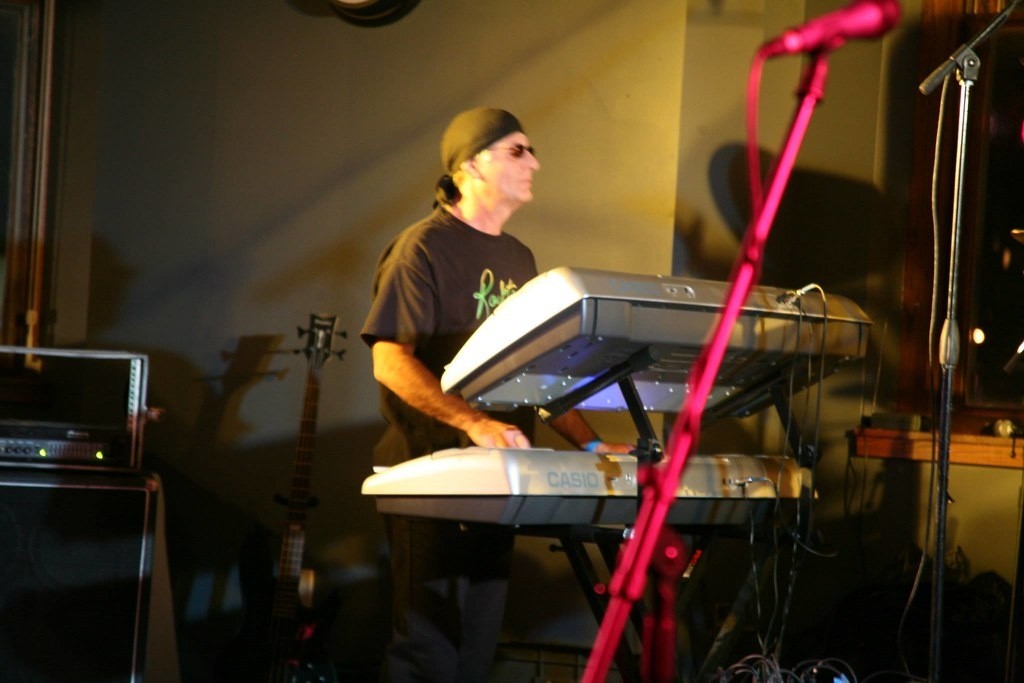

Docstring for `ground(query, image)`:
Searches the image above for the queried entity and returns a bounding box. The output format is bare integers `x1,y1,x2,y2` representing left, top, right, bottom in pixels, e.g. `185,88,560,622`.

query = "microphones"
761,0,903,60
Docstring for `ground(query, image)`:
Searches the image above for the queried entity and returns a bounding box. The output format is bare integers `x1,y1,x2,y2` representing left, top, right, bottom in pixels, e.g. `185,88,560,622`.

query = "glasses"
485,144,536,159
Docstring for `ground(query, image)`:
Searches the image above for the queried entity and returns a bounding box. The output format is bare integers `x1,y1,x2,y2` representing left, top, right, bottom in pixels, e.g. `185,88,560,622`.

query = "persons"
359,107,637,683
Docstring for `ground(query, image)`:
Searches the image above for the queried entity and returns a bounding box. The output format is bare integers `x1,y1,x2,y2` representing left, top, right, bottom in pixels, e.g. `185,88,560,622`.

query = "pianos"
440,266,874,420
360,446,802,683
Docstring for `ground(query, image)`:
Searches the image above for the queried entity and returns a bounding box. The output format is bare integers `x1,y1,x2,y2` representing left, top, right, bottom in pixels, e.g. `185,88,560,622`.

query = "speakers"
0,464,162,683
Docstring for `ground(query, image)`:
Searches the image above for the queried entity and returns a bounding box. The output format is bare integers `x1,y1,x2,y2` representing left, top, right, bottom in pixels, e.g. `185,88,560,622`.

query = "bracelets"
585,438,602,451
